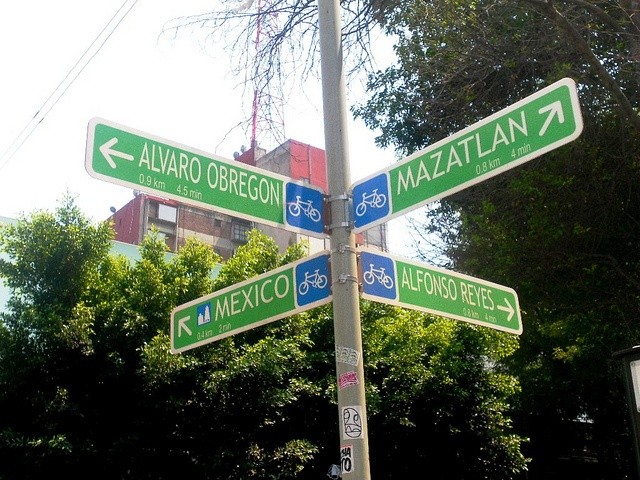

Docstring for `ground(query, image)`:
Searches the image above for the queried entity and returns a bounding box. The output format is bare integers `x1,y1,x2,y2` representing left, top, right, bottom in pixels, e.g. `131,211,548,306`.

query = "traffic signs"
84,117,329,241
357,244,524,335
348,76,585,233
171,249,333,354
